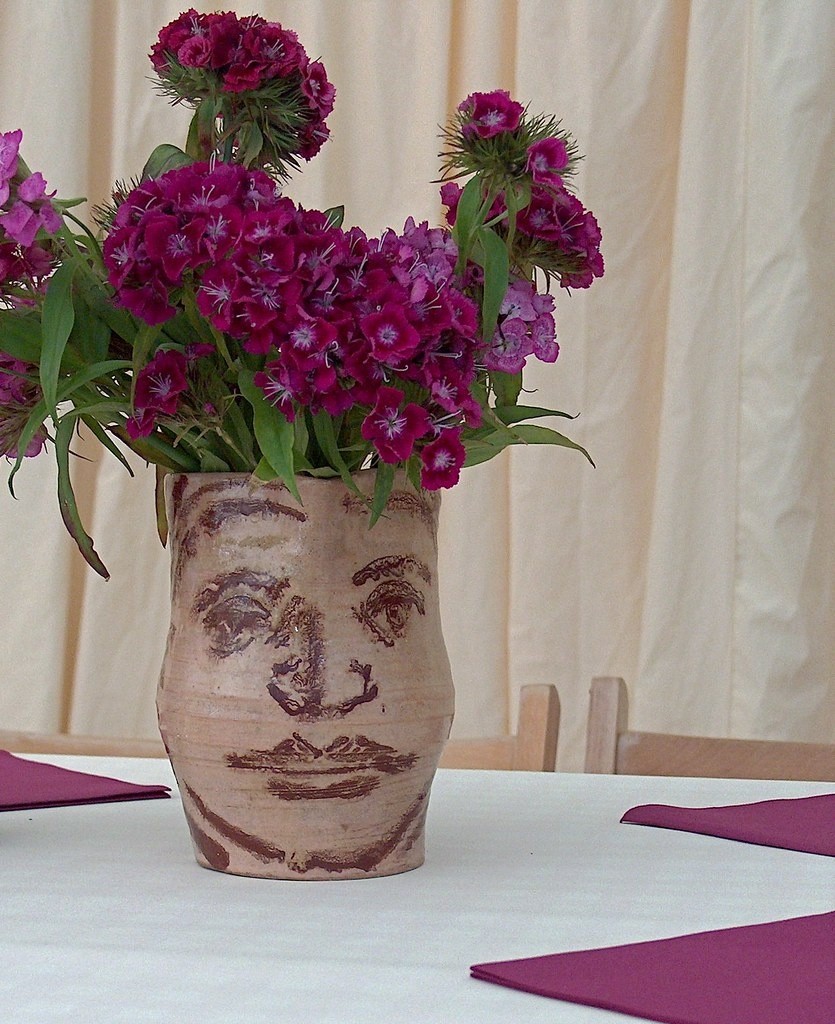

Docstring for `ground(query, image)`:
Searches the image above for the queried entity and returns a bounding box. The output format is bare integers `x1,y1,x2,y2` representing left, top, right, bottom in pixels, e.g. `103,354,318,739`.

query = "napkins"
0,751,171,814
470,908,835,1024
620,792,835,857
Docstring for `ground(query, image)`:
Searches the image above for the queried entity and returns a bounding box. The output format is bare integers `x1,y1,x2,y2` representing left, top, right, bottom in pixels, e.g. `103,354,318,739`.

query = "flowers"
0,9,606,586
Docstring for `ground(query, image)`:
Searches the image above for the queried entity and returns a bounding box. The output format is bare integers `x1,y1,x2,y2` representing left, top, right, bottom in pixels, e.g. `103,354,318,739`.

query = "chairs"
434,675,835,780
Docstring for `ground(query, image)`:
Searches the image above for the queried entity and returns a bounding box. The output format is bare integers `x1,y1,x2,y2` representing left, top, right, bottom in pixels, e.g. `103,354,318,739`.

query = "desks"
1,749,835,1023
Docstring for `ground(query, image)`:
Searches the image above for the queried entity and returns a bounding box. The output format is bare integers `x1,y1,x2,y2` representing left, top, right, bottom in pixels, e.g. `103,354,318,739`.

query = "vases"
154,472,458,881
